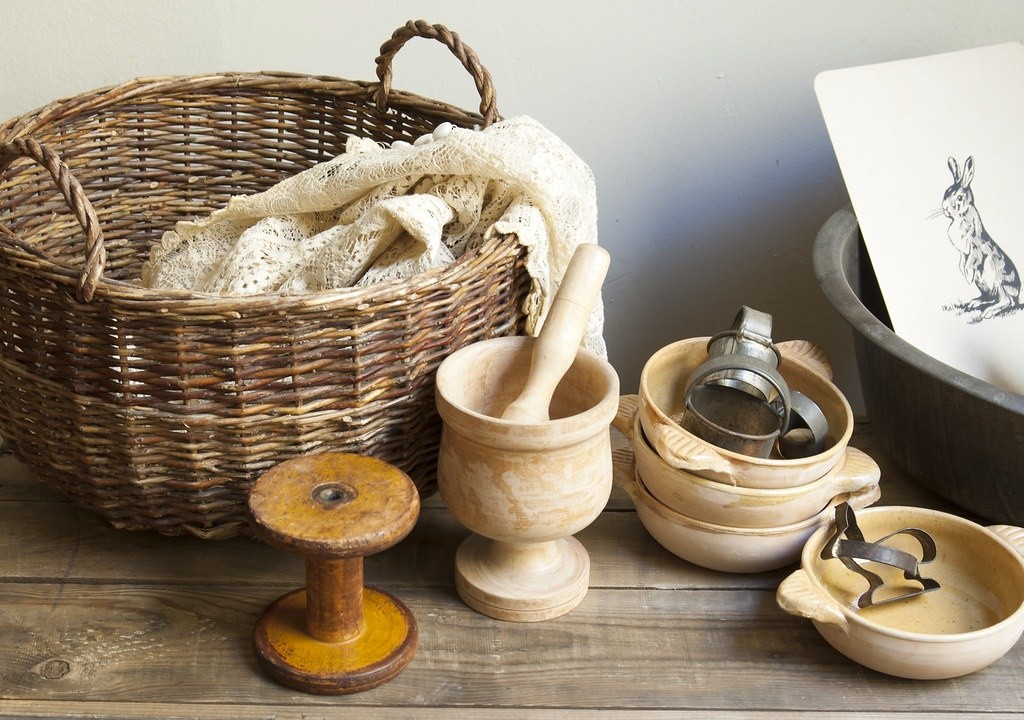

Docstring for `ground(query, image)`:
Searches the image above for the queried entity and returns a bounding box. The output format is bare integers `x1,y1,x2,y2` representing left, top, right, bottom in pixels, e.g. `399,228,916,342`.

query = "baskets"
1,18,536,543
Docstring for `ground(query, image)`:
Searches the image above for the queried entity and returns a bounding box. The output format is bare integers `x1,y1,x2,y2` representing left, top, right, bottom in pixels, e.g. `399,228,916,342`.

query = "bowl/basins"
775,506,1024,680
611,394,881,527
812,204,1024,513
639,340,853,488
612,446,880,572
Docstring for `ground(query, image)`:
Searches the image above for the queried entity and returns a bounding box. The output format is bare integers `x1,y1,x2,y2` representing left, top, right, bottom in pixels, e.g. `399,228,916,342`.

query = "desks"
0,400,1024,720
